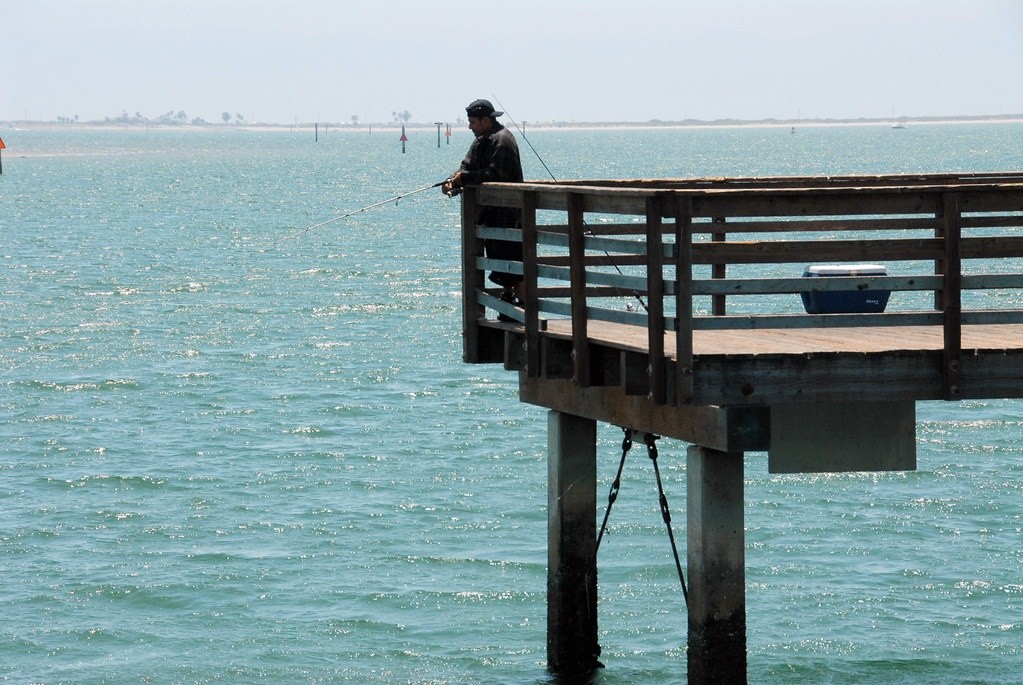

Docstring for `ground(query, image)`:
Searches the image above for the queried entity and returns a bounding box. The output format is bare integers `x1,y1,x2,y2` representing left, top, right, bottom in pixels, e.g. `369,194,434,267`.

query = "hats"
466,99,504,117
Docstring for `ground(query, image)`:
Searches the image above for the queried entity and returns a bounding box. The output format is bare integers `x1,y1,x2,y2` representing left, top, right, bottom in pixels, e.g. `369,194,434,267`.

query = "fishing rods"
492,94,668,336
263,176,463,245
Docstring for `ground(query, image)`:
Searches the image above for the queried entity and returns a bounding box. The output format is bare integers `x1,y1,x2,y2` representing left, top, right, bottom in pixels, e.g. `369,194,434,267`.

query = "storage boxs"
800,265,892,314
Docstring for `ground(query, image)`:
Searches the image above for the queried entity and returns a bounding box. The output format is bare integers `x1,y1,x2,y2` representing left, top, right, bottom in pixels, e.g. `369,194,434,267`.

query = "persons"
442,100,523,321
